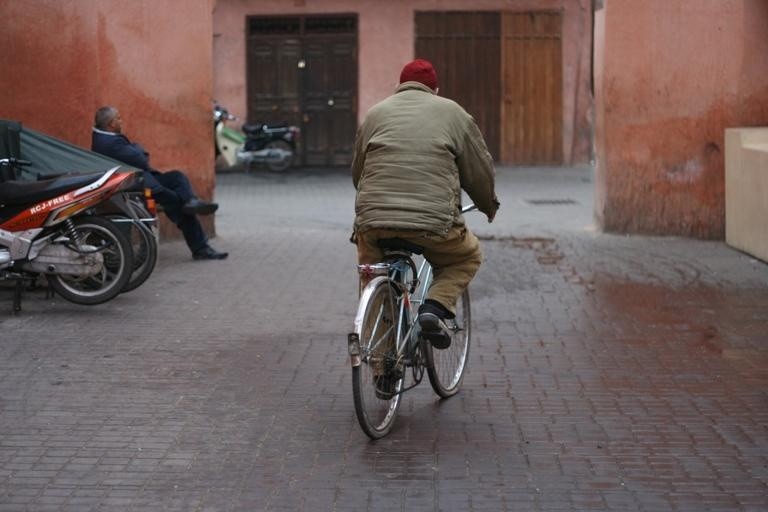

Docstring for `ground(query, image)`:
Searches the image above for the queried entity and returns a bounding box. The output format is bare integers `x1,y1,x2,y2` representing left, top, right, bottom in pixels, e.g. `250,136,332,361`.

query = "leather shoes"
182,197,219,216
193,243,228,261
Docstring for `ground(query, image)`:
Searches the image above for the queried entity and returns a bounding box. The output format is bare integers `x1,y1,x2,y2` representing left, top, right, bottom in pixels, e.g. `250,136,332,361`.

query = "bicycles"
346,204,482,439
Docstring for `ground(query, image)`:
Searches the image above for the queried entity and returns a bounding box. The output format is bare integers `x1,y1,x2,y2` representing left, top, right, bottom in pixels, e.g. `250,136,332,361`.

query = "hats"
400,59,438,91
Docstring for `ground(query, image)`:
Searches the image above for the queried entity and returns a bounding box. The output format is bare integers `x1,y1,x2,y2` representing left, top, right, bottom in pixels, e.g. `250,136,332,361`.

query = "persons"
350,57,499,401
91,105,228,260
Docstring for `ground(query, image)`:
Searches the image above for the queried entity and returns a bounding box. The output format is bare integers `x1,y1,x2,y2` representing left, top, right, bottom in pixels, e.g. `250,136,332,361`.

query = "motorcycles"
212,100,302,176
0,118,160,315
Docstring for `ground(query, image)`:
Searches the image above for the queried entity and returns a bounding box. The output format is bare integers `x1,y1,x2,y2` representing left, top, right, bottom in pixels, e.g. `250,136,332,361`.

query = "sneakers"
418,302,452,349
374,375,395,400
